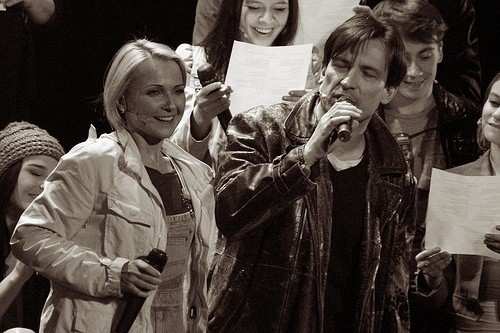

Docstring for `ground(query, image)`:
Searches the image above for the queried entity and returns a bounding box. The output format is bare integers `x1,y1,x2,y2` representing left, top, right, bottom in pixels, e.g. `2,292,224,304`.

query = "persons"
205,15,419,333
0,0,88,157
10,38,218,333
0,121,66,328
410,75,500,333
170,0,314,165
371,0,480,210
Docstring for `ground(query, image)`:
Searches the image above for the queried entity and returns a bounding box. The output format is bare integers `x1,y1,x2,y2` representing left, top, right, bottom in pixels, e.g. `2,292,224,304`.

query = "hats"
0,121,65,175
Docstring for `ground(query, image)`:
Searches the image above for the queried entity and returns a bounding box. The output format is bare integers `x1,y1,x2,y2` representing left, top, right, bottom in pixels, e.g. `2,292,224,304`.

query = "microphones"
197,63,233,136
337,95,358,142
124,111,151,123
477,117,482,125
433,79,439,84
115,248,168,333
319,81,327,98
239,27,249,39
